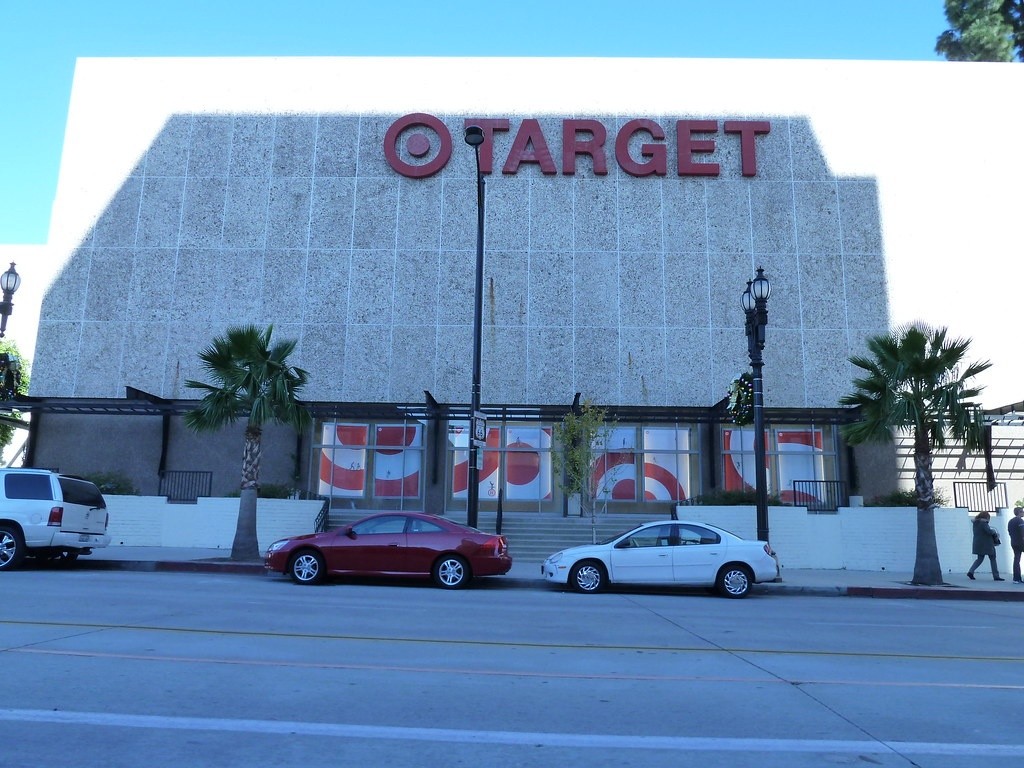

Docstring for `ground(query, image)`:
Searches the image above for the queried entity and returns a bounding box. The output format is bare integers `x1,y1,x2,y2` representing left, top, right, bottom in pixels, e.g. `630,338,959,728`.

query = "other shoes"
967,572,976,580
1013,579,1024,584
994,577,1005,581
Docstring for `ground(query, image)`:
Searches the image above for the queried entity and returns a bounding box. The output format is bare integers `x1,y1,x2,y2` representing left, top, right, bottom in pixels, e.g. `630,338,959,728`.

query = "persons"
1008,507,1024,584
967,511,1005,581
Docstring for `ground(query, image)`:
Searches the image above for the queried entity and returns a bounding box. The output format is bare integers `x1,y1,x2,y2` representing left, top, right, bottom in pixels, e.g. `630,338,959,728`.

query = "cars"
0,467,112,571
541,520,777,599
265,512,512,590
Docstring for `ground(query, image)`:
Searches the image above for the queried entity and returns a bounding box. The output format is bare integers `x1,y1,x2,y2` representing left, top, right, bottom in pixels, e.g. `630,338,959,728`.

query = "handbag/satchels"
992,532,1001,545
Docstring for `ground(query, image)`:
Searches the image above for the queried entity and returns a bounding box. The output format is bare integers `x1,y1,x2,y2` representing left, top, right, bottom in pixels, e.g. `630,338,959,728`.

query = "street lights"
464,124,486,529
740,265,783,584
0,260,21,338
